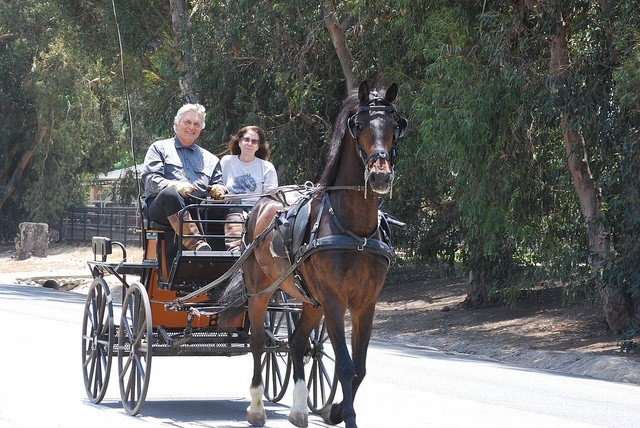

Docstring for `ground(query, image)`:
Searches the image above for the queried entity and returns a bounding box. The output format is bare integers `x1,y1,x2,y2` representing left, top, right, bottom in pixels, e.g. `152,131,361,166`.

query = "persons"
220,125,279,207
140,103,244,251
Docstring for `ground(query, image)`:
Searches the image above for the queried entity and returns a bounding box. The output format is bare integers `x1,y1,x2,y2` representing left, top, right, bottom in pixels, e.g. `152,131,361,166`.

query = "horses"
213,79,409,427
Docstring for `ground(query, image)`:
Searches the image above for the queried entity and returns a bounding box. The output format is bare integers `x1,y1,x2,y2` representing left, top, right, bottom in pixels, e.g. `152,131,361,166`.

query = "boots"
167,208,212,251
224,213,245,251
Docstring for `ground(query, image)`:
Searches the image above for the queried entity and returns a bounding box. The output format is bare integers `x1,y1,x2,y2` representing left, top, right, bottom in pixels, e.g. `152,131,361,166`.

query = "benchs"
140,197,180,291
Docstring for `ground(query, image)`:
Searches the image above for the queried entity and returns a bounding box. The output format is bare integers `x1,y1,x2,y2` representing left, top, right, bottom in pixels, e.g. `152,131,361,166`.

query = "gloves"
176,181,197,198
207,183,227,200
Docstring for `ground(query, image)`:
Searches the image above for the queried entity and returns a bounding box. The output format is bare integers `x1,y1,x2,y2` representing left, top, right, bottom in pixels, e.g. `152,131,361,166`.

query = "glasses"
244,137,258,144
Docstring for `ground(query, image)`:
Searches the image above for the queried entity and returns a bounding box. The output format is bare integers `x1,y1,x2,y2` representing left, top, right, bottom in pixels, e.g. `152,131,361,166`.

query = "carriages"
81,80,409,428
